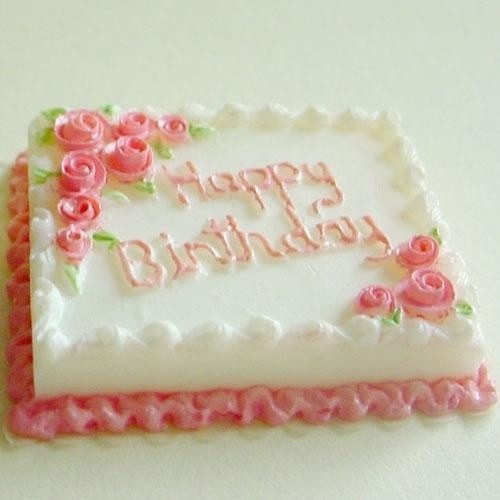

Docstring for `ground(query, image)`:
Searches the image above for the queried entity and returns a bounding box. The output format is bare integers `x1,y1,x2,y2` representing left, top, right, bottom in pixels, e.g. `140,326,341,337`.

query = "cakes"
6,100,498,442
